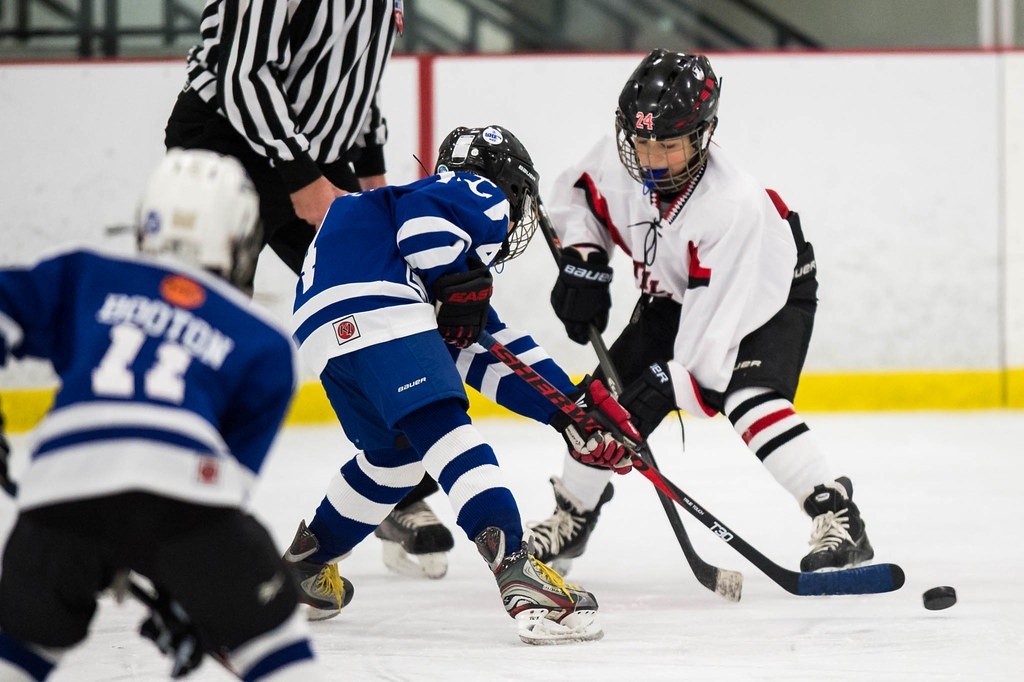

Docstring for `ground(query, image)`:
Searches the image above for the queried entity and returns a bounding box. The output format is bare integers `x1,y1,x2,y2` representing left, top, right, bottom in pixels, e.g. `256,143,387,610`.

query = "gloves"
141,594,203,678
551,246,613,344
434,258,493,350
611,356,679,441
549,374,646,475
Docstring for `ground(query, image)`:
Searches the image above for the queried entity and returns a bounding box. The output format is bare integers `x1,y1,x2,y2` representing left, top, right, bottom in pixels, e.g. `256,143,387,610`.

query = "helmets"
617,47,721,195
138,149,261,277
434,125,540,265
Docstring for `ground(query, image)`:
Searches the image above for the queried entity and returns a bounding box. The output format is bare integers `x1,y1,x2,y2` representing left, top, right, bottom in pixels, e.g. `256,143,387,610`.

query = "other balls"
924,584,958,612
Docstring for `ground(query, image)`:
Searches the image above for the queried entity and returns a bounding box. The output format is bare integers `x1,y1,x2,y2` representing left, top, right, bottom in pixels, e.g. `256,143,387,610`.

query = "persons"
524,49,876,577
284,125,642,646
164,0,454,578
0,151,315,682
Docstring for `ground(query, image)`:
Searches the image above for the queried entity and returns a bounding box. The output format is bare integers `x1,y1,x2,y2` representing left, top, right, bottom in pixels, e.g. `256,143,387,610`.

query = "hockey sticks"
585,325,744,603
3,478,236,679
476,329,907,597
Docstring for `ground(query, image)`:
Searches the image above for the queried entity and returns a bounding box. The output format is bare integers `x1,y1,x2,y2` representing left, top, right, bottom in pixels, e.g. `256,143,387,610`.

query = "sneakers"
476,526,604,644
800,476,877,573
374,499,455,580
521,476,615,576
281,520,354,621
